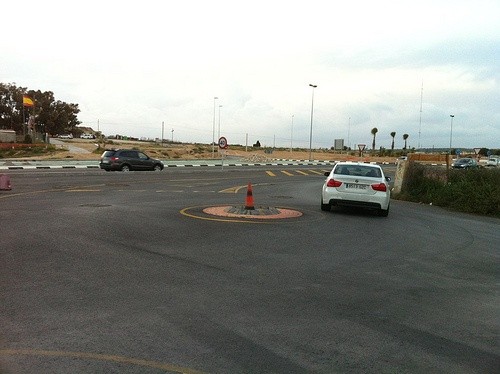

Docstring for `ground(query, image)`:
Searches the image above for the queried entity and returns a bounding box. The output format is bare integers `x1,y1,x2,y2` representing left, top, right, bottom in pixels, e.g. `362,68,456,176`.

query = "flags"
23,96,34,107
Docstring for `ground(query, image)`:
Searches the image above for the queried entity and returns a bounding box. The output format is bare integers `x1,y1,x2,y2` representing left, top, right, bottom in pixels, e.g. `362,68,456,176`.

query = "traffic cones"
244,180,255,210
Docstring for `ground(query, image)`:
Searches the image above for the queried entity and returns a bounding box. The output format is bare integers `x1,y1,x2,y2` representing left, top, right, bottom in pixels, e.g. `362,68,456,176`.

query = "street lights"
307,83,317,160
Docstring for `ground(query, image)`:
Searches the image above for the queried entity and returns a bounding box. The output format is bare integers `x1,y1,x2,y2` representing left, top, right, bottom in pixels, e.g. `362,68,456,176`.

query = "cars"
451,158,481,170
320,162,390,217
59,133,96,139
99,148,164,172
487,158,500,167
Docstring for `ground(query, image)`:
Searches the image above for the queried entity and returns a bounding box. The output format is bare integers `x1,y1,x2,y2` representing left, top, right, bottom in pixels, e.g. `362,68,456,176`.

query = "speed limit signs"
219,137,227,149
